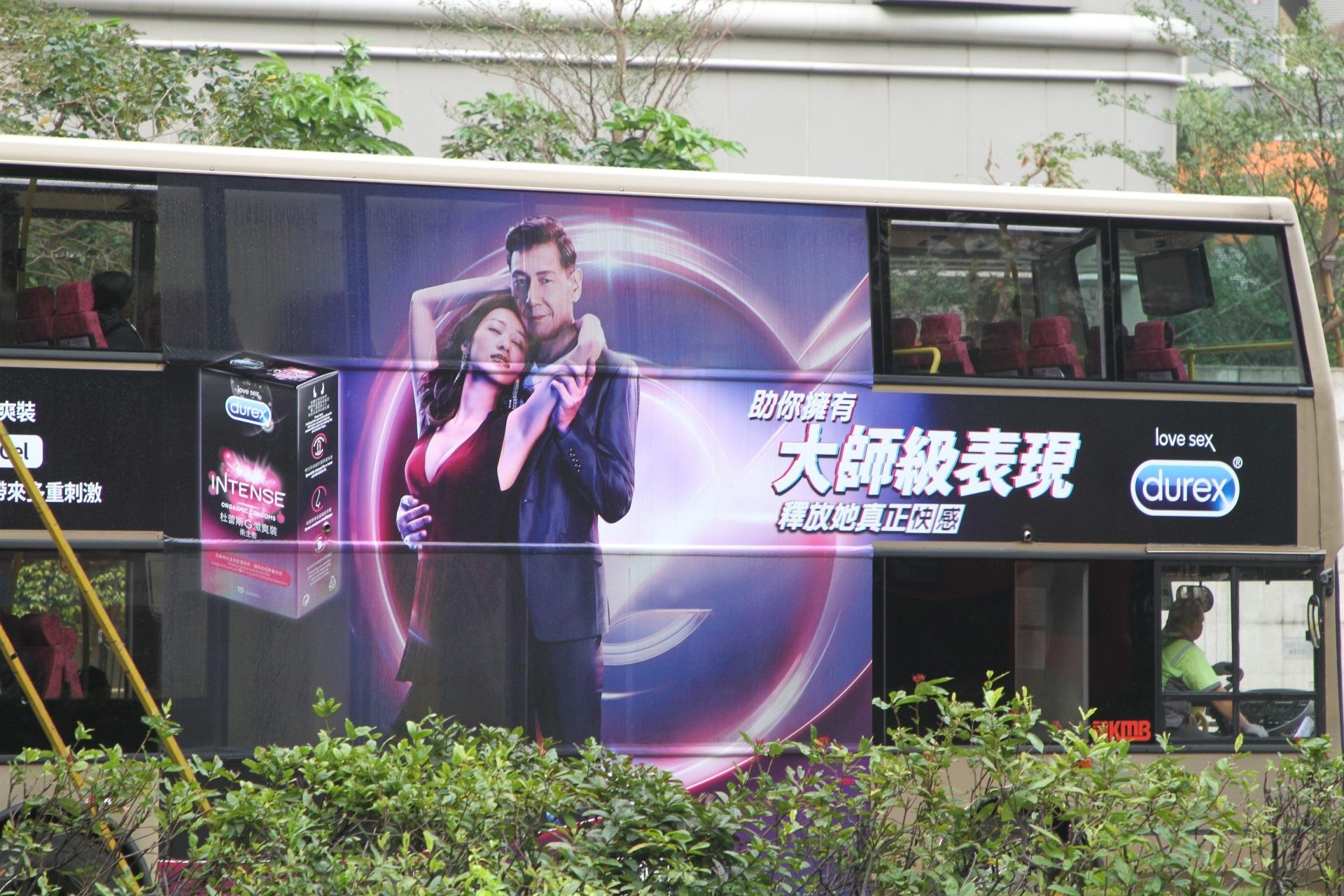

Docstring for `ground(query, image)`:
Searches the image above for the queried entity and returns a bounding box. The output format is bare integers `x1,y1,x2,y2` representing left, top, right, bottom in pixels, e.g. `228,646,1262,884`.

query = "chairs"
892,312,1188,385
18,282,106,349
0,608,82,699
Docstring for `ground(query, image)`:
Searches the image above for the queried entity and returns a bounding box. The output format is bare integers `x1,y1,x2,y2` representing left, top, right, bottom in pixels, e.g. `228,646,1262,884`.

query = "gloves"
1242,721,1269,738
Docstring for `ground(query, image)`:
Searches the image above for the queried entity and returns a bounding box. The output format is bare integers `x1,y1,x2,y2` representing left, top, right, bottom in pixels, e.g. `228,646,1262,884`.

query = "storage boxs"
201,349,340,620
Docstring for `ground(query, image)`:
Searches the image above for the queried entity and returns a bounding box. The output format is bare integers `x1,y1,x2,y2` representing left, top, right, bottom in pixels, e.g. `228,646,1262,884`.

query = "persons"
91,270,145,352
388,274,605,745
507,215,634,766
1160,598,1269,738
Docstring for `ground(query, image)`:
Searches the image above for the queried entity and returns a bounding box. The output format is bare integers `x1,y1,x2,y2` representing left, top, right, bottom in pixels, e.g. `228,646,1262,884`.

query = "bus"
0,128,1343,896
